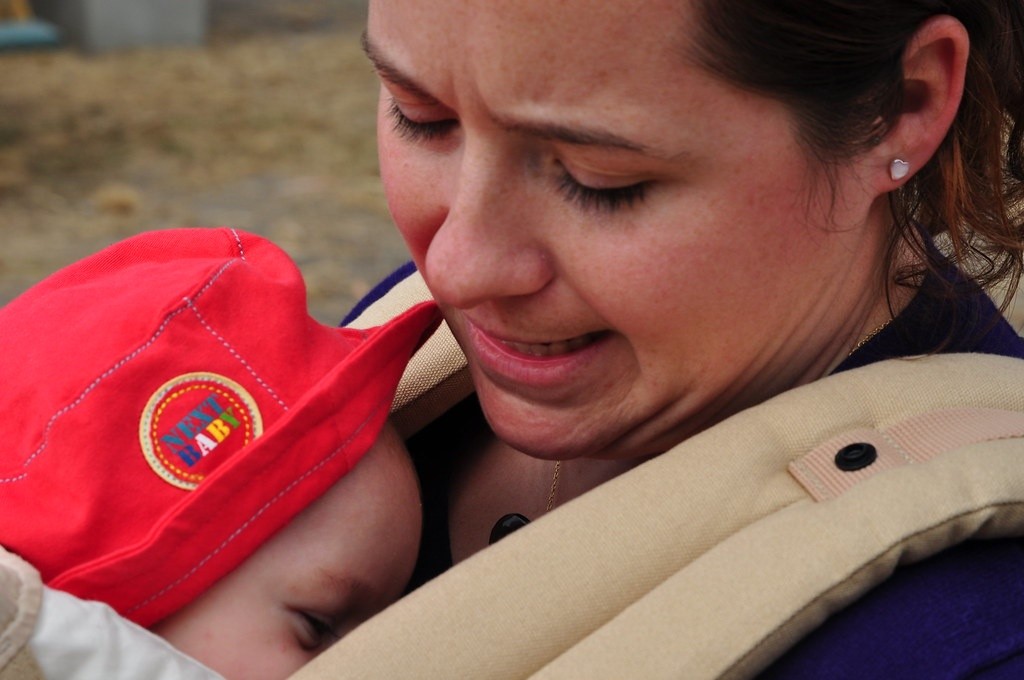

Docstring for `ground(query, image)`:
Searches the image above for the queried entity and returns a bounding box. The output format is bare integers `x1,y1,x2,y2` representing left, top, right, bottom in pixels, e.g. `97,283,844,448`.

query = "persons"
0,0,1024,680
0,223,444,680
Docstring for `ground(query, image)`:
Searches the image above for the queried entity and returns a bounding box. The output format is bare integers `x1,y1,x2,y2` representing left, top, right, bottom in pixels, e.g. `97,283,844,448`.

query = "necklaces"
546,459,562,512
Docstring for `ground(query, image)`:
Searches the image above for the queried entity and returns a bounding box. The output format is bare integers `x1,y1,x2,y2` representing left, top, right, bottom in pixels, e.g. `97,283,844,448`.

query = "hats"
0,227,446,628
